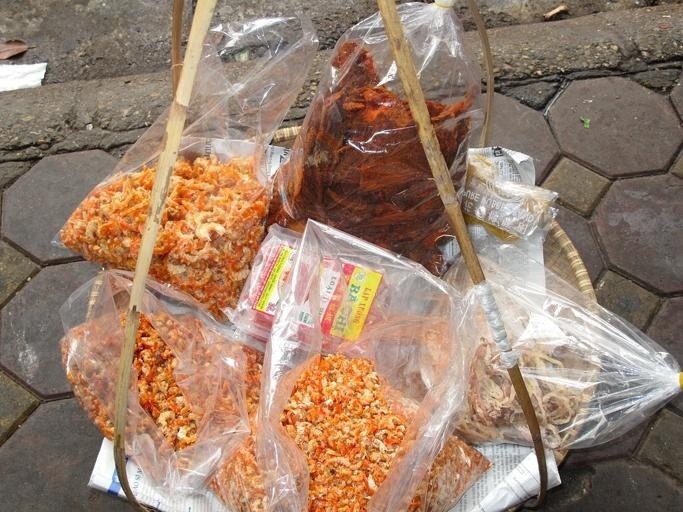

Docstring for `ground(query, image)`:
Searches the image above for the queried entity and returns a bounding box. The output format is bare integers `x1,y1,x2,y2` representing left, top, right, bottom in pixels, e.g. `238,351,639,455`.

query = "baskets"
84,2,599,512
